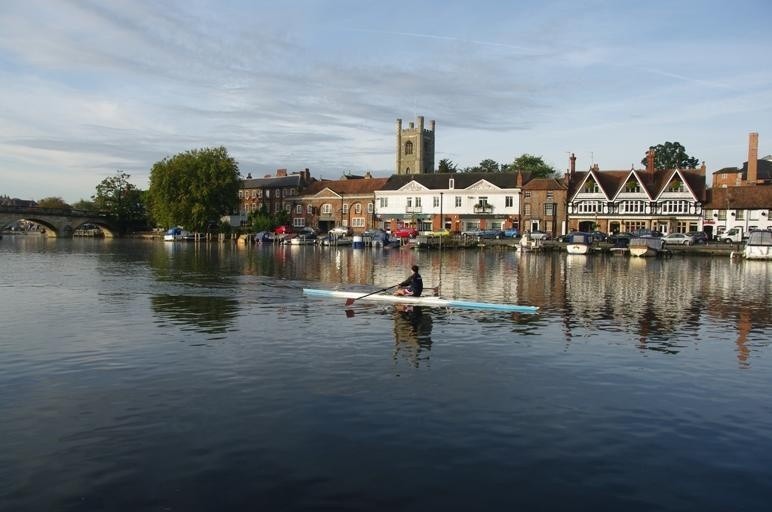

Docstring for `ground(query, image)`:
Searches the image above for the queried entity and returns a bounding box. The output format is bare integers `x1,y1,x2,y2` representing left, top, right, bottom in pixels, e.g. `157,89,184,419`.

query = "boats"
638,258,658,269
563,231,595,255
238,244,343,253
164,241,184,257
237,232,357,244
565,255,589,268
300,285,539,314
163,228,184,241
625,237,660,258
740,228,772,260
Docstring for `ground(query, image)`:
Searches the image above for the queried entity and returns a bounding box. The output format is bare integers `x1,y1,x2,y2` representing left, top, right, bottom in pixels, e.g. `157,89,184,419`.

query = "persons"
394,265,423,297
399,305,423,334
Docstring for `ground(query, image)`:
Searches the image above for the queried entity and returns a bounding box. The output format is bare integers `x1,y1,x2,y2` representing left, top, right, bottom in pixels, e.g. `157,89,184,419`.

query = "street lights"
438,191,445,230
371,199,376,228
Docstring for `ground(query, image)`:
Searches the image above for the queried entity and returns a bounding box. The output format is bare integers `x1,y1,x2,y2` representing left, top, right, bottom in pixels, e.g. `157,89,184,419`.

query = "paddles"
345,285,400,308
421,285,441,291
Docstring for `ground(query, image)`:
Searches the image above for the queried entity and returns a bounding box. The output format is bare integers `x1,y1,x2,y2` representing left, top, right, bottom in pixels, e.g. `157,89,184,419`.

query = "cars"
295,226,321,235
328,226,353,238
390,227,420,239
460,227,746,244
362,229,385,236
428,227,455,238
273,225,292,234
